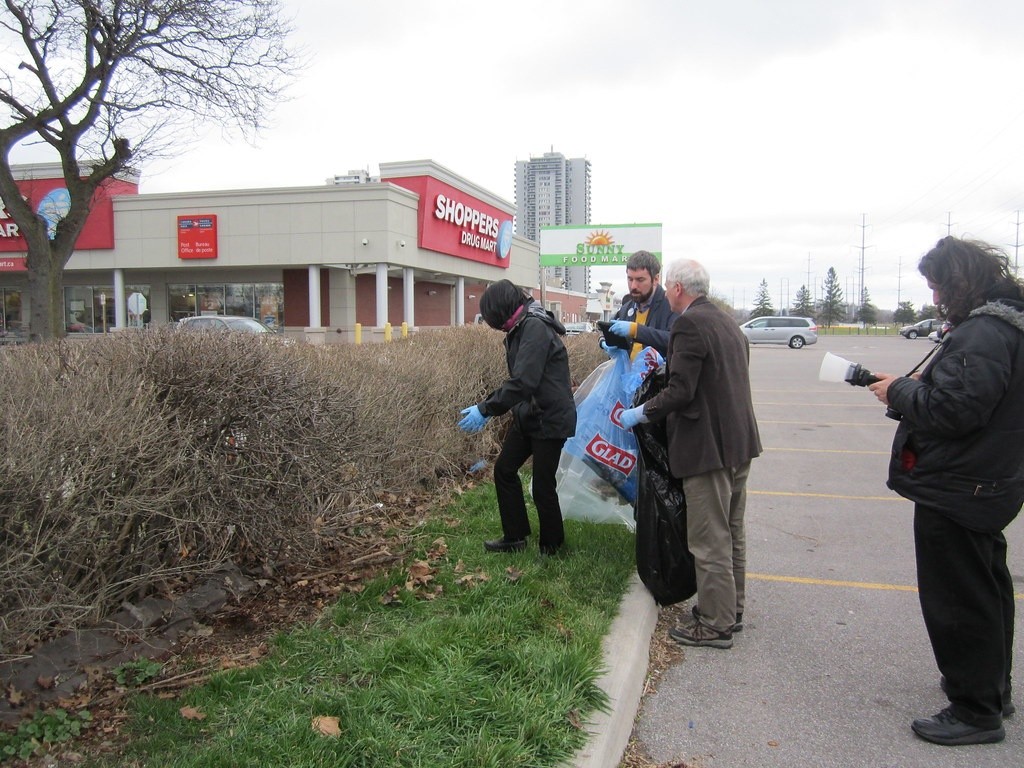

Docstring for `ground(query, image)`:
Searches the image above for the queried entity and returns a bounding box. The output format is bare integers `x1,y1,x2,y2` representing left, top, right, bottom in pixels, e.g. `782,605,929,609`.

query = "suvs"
175,315,296,345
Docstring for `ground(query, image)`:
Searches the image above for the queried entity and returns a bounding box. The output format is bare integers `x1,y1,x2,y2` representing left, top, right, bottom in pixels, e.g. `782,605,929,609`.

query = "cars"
928,321,951,343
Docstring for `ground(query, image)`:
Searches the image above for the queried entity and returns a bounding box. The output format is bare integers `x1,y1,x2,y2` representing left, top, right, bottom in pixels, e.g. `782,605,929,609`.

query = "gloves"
601,341,617,357
608,320,630,338
469,461,486,473
620,409,639,431
457,405,491,434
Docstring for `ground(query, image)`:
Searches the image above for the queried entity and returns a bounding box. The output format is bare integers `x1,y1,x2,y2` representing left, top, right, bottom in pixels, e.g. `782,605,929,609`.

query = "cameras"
818,352,904,421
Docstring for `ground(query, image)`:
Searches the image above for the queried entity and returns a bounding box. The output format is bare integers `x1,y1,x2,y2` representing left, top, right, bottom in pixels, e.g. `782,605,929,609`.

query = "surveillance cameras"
362,239,368,245
400,241,405,248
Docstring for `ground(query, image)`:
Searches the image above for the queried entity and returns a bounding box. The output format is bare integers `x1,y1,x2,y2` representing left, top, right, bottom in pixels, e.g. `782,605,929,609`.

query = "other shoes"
540,545,558,556
484,537,527,553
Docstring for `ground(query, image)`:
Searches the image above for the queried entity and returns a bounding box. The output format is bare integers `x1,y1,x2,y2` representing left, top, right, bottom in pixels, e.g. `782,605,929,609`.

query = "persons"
594,252,675,365
457,278,578,555
869,235,1024,746
621,259,762,650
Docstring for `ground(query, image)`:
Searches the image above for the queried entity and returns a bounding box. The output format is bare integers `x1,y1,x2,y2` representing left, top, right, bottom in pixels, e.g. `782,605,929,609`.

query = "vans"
899,319,942,339
738,316,818,349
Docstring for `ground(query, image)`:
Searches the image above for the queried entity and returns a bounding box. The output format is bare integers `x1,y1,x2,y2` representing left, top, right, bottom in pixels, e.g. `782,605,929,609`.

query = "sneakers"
940,676,1016,716
911,709,1006,746
668,620,734,649
692,605,743,631
